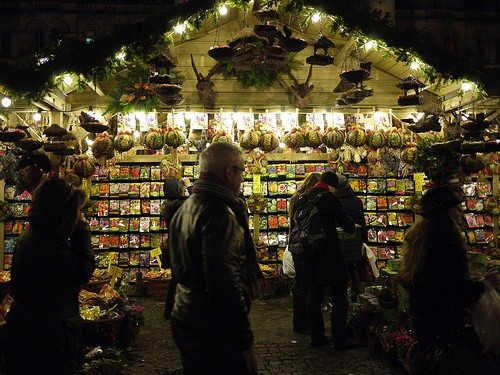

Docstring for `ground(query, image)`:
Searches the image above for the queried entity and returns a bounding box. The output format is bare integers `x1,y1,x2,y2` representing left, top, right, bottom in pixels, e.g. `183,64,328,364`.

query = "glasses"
232,166,248,176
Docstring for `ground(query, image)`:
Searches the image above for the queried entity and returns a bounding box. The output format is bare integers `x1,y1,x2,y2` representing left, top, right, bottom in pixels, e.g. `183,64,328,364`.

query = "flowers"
367,317,413,360
132,304,144,321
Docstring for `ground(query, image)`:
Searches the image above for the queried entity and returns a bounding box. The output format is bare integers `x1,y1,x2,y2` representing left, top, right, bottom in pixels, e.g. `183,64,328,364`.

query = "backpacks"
288,190,331,255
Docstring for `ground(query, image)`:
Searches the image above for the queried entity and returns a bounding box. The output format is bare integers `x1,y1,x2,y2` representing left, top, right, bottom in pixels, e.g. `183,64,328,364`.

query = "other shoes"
333,339,362,350
311,336,333,347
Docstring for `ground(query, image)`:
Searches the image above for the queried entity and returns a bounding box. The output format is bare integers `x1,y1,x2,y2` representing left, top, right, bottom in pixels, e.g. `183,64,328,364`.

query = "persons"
288,170,368,350
397,184,498,375
169,142,265,375
162,177,193,228
5,177,96,375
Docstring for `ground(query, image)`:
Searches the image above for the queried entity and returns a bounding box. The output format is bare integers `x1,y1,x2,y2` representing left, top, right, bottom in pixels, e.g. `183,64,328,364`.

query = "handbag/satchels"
470,276,500,356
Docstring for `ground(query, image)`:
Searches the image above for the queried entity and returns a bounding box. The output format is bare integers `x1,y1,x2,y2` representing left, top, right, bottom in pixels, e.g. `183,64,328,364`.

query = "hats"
420,184,464,216
162,179,184,199
320,171,338,189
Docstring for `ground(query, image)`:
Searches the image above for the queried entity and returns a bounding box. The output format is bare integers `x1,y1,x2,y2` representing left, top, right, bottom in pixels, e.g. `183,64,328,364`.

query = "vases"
366,332,408,375
131,315,144,333
